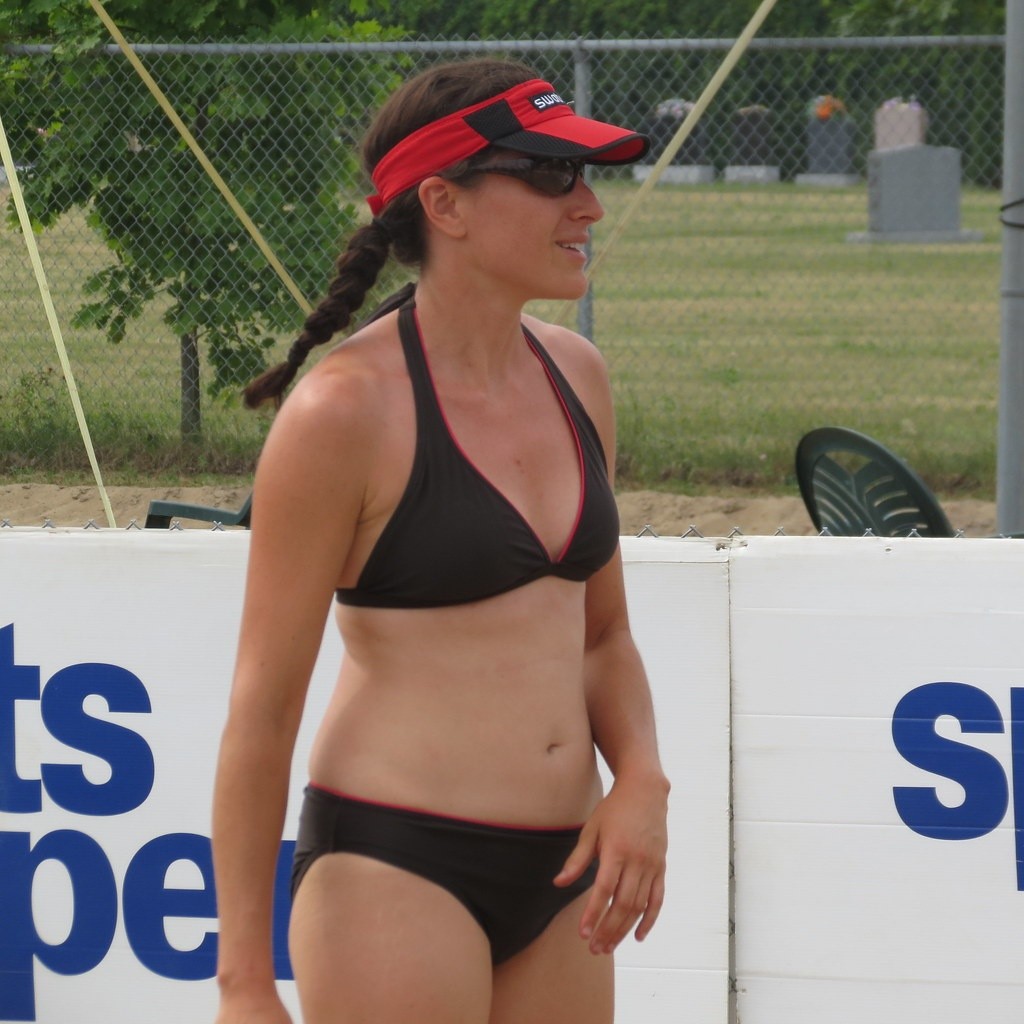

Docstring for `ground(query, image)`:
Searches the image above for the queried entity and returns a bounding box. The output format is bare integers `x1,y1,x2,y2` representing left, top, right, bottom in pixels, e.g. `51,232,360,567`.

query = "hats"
365,79,650,218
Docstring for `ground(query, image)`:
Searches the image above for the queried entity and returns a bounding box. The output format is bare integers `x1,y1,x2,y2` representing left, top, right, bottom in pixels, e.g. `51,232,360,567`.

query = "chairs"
796,425,959,536
145,490,253,529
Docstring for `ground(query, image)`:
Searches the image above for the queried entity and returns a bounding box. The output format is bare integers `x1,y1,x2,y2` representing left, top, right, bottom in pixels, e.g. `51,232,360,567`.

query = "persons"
212,62,672,1024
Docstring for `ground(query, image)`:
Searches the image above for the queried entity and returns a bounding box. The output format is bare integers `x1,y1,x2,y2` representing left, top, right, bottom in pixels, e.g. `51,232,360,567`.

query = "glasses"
466,156,586,195
